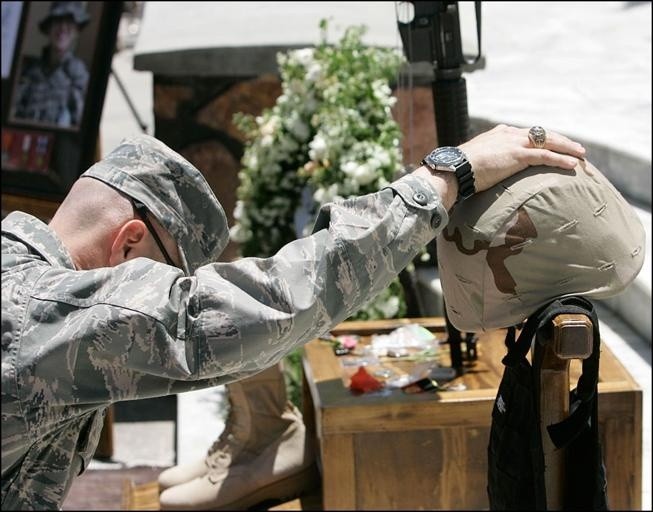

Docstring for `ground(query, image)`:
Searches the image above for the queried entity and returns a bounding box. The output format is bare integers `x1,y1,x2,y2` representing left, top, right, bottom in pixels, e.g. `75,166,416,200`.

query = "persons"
1,124,586,511
13,1,90,128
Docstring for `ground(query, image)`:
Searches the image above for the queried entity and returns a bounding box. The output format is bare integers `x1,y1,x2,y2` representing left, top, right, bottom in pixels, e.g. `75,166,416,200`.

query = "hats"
36,1,93,34
79,134,234,273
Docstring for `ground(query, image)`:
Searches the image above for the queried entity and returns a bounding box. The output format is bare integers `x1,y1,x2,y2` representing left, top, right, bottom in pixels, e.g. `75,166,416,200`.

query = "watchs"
420,143,474,204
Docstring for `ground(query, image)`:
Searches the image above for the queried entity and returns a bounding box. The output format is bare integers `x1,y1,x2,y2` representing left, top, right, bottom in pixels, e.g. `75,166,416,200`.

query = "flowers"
223,14,432,411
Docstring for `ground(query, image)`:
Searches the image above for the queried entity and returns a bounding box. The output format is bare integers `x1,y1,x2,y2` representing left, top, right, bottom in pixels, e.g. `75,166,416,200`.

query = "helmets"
437,154,647,334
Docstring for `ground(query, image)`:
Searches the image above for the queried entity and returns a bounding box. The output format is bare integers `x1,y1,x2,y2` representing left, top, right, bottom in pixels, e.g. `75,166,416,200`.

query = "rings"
527,126,545,150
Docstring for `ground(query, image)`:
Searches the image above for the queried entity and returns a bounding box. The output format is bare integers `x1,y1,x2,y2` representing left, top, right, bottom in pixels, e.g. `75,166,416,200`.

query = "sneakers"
156,374,318,509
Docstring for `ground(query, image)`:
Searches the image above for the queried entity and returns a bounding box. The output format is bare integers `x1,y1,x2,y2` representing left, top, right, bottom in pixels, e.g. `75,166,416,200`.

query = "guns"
396,0,485,376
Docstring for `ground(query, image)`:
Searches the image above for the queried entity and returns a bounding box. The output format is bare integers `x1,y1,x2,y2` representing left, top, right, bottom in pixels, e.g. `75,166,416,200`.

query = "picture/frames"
1,1,125,204
